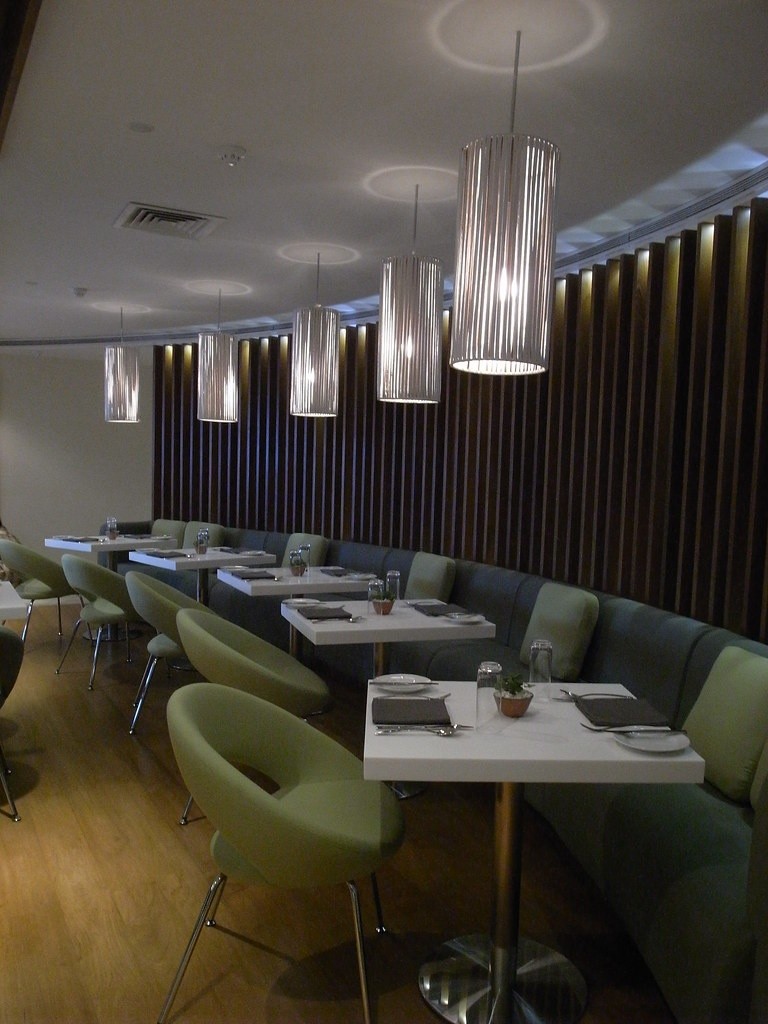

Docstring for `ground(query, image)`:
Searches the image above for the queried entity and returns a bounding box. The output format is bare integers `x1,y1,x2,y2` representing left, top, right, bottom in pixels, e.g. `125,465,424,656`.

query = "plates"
136,548,158,553
283,598,320,607
347,573,377,580
406,598,485,623
241,551,265,556
222,566,247,571
150,535,172,539
613,725,689,752
52,534,75,539
374,674,431,692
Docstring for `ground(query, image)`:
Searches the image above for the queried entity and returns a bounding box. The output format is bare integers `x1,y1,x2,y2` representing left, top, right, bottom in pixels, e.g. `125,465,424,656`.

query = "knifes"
603,729,687,735
282,601,326,604
553,698,576,702
369,681,439,686
374,725,474,729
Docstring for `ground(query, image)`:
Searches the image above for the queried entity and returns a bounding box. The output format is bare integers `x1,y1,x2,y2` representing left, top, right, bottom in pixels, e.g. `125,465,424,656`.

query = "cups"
106,517,117,543
368,581,382,616
387,571,400,604
299,544,310,573
198,528,208,555
529,640,552,703
476,662,502,734
290,551,301,580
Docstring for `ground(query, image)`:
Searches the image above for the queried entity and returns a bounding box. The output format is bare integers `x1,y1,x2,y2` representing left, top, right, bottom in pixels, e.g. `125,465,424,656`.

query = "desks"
362,671,706,1024
47,533,149,644
125,545,273,666
210,571,378,679
277,598,495,803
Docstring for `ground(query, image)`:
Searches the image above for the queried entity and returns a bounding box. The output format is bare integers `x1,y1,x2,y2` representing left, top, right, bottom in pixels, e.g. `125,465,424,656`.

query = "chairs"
0,532,410,1024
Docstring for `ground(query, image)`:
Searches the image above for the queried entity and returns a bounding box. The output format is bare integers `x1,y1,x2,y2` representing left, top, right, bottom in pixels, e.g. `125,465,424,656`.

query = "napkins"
369,694,453,729
415,603,467,619
221,548,266,553
299,609,352,622
145,551,187,558
570,696,671,731
123,534,152,539
231,569,280,580
65,536,99,546
319,567,370,576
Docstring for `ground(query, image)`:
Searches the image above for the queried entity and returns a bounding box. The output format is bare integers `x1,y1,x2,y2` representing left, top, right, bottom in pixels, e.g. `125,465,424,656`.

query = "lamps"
105,30,558,422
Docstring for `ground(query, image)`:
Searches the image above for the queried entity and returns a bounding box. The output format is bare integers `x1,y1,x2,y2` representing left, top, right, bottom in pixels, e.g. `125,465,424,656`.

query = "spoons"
374,724,459,736
559,689,633,699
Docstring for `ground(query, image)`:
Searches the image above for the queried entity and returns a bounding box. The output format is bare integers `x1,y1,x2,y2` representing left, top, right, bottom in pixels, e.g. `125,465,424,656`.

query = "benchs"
97,519,768,1024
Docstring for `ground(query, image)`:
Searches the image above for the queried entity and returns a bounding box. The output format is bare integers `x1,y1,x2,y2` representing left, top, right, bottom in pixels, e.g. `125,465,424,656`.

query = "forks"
579,722,677,732
374,693,451,700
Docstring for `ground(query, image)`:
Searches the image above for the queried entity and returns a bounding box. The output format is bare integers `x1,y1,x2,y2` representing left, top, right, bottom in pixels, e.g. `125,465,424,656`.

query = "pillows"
281,532,330,567
520,582,599,681
402,551,455,605
152,519,228,549
683,645,768,812
